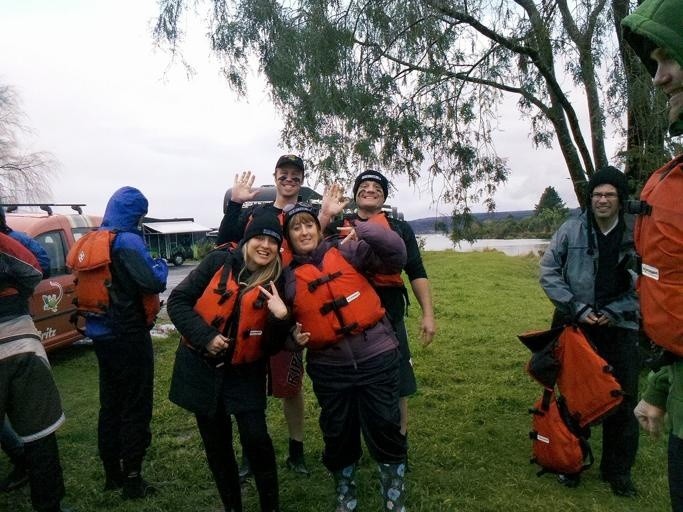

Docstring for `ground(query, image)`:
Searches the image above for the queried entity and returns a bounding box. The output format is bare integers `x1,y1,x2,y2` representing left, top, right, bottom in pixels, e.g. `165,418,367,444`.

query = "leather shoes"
603,473,636,497
2,468,29,492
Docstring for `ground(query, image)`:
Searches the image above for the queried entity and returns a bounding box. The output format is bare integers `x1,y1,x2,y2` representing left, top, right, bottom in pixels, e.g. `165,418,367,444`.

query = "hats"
243,205,284,247
275,154,305,170
588,166,628,255
353,170,388,199
284,203,321,238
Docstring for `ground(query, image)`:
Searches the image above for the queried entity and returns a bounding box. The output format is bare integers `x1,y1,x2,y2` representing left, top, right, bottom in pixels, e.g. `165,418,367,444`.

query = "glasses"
589,193,617,198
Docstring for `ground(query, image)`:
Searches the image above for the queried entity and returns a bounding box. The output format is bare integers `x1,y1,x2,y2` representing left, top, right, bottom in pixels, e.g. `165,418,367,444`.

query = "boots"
332,464,358,512
239,447,250,479
380,463,407,512
286,438,310,475
123,463,155,498
105,464,124,489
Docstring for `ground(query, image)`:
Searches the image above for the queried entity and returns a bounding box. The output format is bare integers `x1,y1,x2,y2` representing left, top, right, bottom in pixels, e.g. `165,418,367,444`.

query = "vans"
0,203,163,354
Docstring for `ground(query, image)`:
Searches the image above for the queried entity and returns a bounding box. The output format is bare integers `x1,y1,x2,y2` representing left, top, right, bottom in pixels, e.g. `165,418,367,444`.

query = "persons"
536,167,643,495
620,0,683,511
1,213,68,511
166,155,437,510
86,186,168,495
